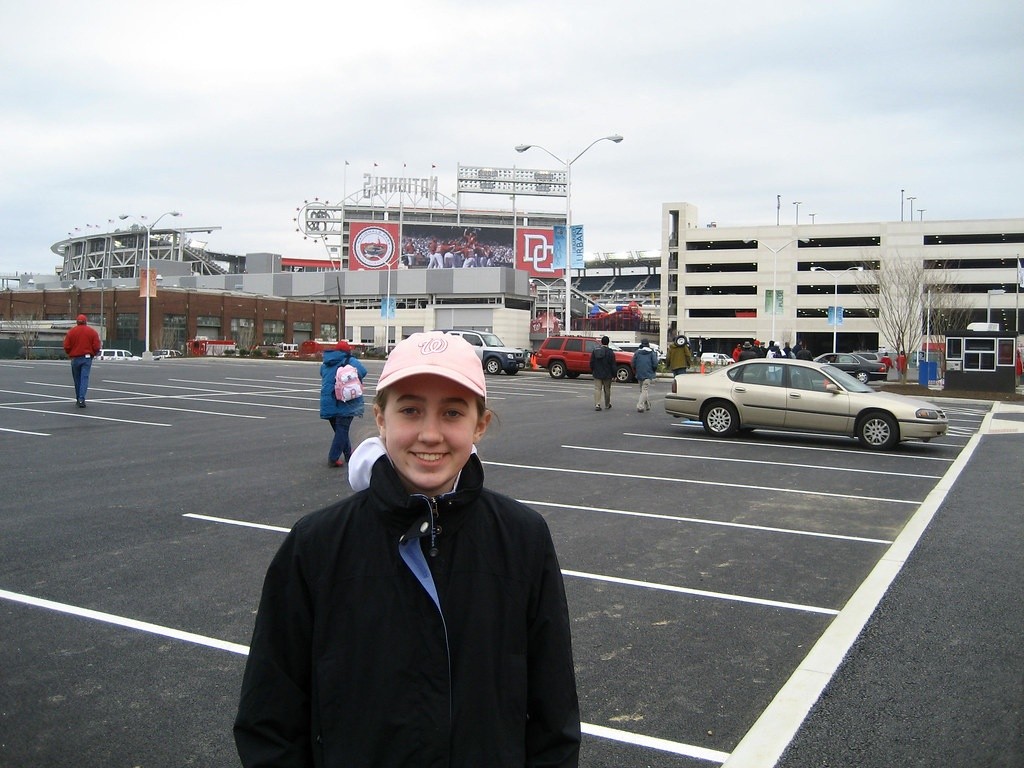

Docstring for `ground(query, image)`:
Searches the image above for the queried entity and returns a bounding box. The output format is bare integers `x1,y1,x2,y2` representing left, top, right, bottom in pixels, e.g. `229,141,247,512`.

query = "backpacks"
335,356,363,401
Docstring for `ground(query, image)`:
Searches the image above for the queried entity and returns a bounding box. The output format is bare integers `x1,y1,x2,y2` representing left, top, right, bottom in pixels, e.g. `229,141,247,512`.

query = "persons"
632,339,657,412
1017,350,1022,386
897,351,908,385
320,341,367,467
829,356,835,363
880,352,893,381
63,315,101,407
733,340,814,362
234,332,582,768
401,229,514,269
667,337,691,377
590,336,616,411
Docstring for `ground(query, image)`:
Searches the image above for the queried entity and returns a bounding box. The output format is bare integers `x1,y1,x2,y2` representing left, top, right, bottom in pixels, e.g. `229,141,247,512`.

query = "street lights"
916,210,926,221
986,289,1005,322
514,134,623,333
792,201,804,224
743,239,812,341
366,254,415,357
88,277,105,348
117,210,181,360
808,213,819,223
528,278,567,341
810,266,864,353
906,196,916,222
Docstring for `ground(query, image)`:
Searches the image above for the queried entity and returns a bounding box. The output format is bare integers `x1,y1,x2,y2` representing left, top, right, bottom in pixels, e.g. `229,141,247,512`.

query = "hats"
77,315,87,322
378,331,487,407
641,339,649,348
754,341,760,345
335,341,355,351
742,342,752,347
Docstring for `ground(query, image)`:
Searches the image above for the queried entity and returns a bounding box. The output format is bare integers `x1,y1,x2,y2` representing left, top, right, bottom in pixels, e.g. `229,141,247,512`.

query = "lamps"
88,276,95,282
157,274,163,280
28,279,33,283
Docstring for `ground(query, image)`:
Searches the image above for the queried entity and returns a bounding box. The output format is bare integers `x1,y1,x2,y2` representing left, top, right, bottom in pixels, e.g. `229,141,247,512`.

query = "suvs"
94,348,143,360
152,349,181,359
534,334,637,383
445,330,526,374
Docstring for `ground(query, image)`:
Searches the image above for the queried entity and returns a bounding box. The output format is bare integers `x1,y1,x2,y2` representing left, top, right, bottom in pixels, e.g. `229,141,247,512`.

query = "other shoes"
76,400,86,408
606,403,612,410
329,461,343,467
595,403,602,412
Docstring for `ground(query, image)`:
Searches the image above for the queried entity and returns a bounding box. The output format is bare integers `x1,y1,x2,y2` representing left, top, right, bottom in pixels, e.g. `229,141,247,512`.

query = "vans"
611,343,667,364
700,352,735,366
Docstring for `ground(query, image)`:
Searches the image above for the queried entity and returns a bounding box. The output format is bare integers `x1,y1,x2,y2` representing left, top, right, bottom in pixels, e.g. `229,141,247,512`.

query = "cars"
801,353,888,386
664,358,949,451
855,352,882,364
